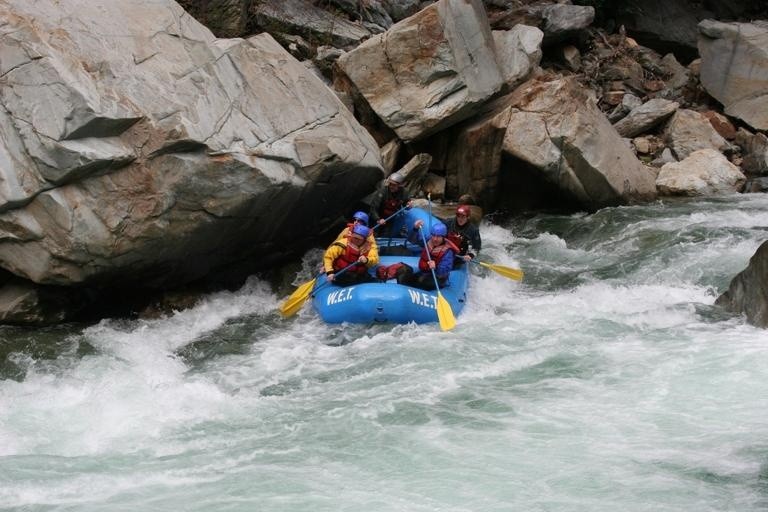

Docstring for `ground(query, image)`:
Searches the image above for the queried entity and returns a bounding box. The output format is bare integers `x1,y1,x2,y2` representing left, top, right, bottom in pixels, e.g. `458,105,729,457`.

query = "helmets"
352,225,370,239
352,211,369,224
387,172,405,186
430,222,448,237
455,204,472,219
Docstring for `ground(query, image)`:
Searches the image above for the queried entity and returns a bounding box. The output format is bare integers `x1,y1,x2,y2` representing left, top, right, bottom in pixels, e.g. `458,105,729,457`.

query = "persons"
396,219,454,293
444,202,481,269
324,224,379,287
367,172,414,235
319,211,377,273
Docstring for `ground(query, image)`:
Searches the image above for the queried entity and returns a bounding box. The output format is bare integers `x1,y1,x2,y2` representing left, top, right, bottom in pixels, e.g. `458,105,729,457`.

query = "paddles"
457,255,524,282
418,224,457,332
280,255,361,316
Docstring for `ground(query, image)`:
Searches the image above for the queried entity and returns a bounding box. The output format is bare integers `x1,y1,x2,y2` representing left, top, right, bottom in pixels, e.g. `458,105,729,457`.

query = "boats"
312,207,469,325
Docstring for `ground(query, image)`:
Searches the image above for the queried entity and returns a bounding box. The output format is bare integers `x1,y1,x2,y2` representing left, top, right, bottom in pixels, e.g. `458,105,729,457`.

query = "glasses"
354,218,361,222
